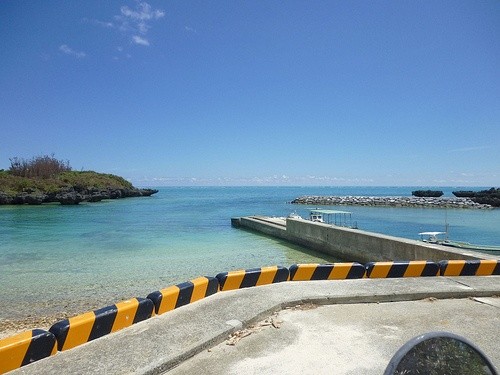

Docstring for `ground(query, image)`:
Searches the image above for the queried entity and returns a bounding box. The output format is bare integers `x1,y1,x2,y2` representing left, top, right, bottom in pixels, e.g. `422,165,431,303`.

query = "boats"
411,189,443,197
418,230,500,255
308,208,359,230
451,190,474,196
